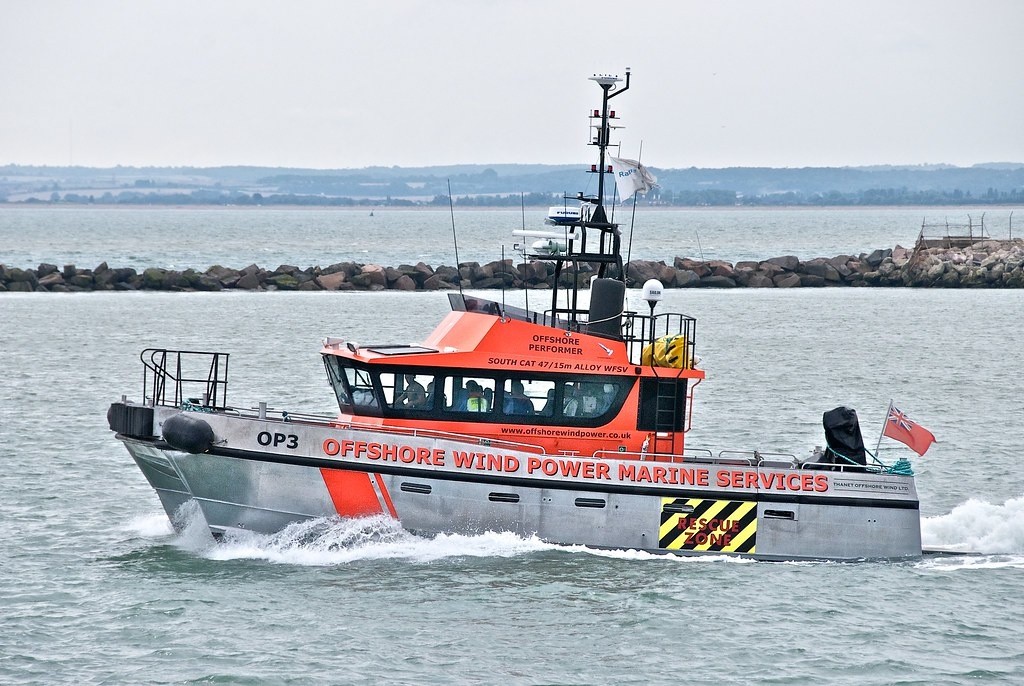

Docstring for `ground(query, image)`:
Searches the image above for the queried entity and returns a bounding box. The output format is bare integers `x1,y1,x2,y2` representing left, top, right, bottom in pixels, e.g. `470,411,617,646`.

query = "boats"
107,69,937,567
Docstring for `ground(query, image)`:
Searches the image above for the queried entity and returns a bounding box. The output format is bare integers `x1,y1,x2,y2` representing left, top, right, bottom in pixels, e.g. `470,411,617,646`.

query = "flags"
882,405,937,456
608,152,662,203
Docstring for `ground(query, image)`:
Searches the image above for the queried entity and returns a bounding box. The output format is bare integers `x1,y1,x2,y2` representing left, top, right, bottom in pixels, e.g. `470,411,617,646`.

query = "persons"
394,374,615,418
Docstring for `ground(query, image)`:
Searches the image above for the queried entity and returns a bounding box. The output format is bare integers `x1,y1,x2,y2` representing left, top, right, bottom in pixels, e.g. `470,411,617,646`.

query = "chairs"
451,387,555,416
